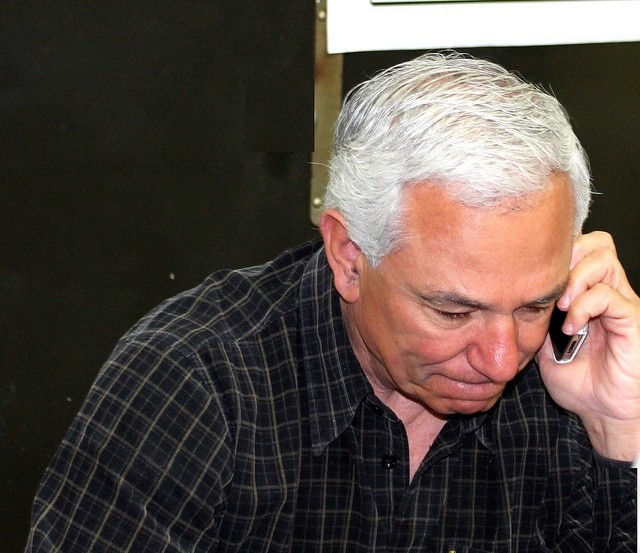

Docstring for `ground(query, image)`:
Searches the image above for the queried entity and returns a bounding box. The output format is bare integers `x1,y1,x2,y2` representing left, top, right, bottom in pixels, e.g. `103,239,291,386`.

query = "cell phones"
548,301,589,364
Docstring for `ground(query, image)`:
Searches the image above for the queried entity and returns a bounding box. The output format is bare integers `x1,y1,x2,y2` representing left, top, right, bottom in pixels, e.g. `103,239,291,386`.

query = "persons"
22,53,640,552
